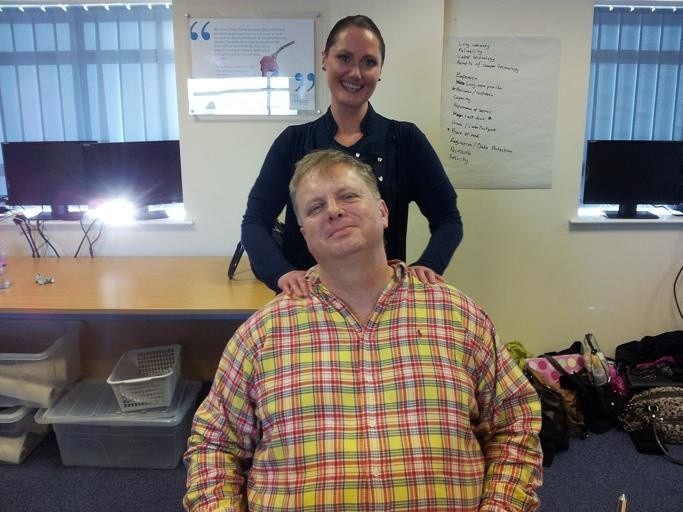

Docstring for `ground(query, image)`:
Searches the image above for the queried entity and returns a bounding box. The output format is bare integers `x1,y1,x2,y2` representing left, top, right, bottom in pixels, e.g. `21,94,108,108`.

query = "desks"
1,255,279,384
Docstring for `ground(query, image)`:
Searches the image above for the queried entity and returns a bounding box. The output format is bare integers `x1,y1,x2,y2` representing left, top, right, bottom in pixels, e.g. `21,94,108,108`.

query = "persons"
182,150,543,512
241,15,463,298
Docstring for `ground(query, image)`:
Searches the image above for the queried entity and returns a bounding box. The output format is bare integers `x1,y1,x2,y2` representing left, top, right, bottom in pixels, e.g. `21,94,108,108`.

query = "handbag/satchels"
503,331,682,466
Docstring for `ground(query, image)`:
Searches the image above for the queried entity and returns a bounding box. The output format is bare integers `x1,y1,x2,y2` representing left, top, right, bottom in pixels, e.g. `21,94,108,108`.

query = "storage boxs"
1,315,206,472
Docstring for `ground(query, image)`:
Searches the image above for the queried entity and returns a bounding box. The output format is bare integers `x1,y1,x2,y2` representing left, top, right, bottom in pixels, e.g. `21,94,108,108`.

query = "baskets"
107,345,181,412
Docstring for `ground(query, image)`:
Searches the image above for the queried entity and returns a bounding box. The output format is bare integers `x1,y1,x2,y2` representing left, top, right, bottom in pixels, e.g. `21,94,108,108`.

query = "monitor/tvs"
583,140,682,218
80,140,183,220
1,140,97,220
